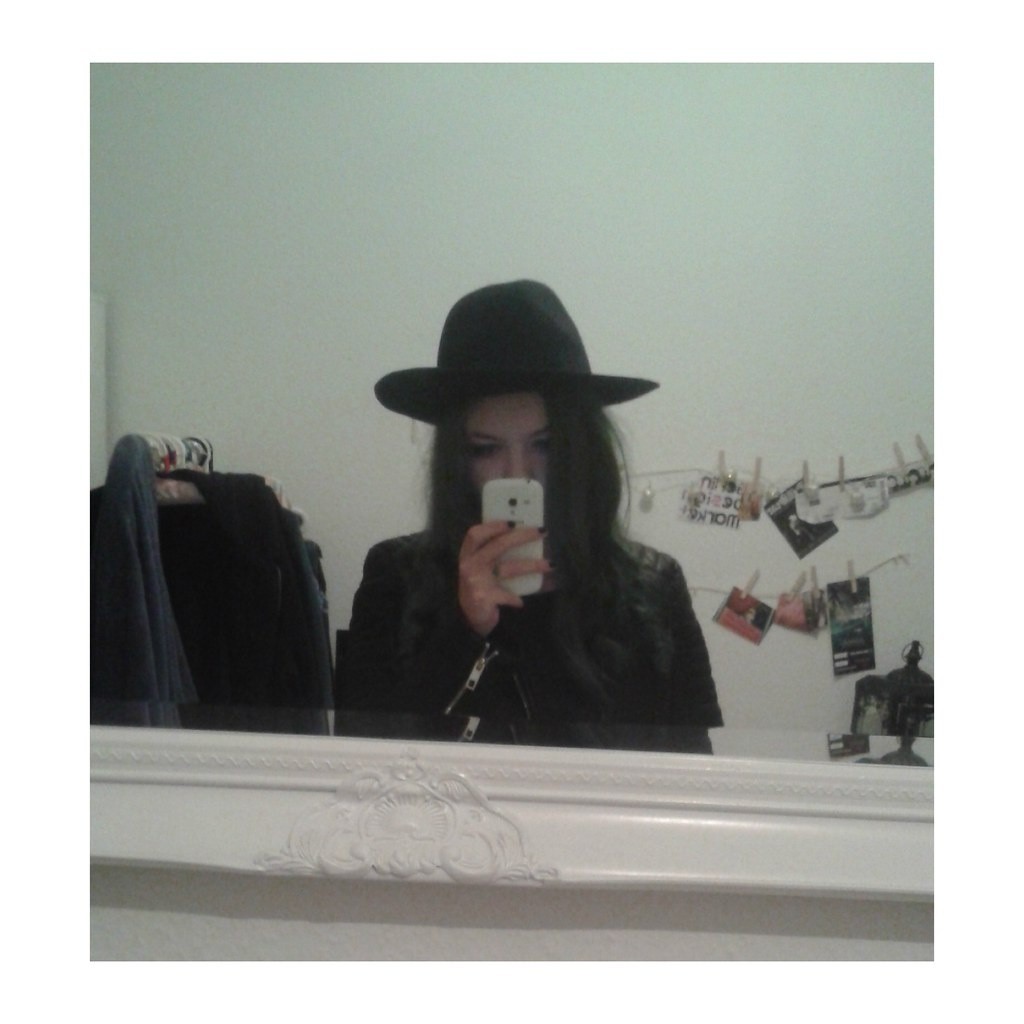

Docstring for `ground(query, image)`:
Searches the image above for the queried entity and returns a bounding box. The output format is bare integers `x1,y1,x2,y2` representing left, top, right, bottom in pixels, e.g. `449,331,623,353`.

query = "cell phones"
482,477,545,596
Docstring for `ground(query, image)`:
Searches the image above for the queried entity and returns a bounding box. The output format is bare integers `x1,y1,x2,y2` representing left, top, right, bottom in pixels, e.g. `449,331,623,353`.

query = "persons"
337,281,726,753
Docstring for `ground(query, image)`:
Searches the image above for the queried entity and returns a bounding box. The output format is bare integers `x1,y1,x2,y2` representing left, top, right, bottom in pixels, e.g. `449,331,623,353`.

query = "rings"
491,563,502,585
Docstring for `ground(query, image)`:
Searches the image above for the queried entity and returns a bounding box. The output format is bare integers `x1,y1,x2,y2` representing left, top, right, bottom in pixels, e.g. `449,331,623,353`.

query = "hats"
373,278,661,427
